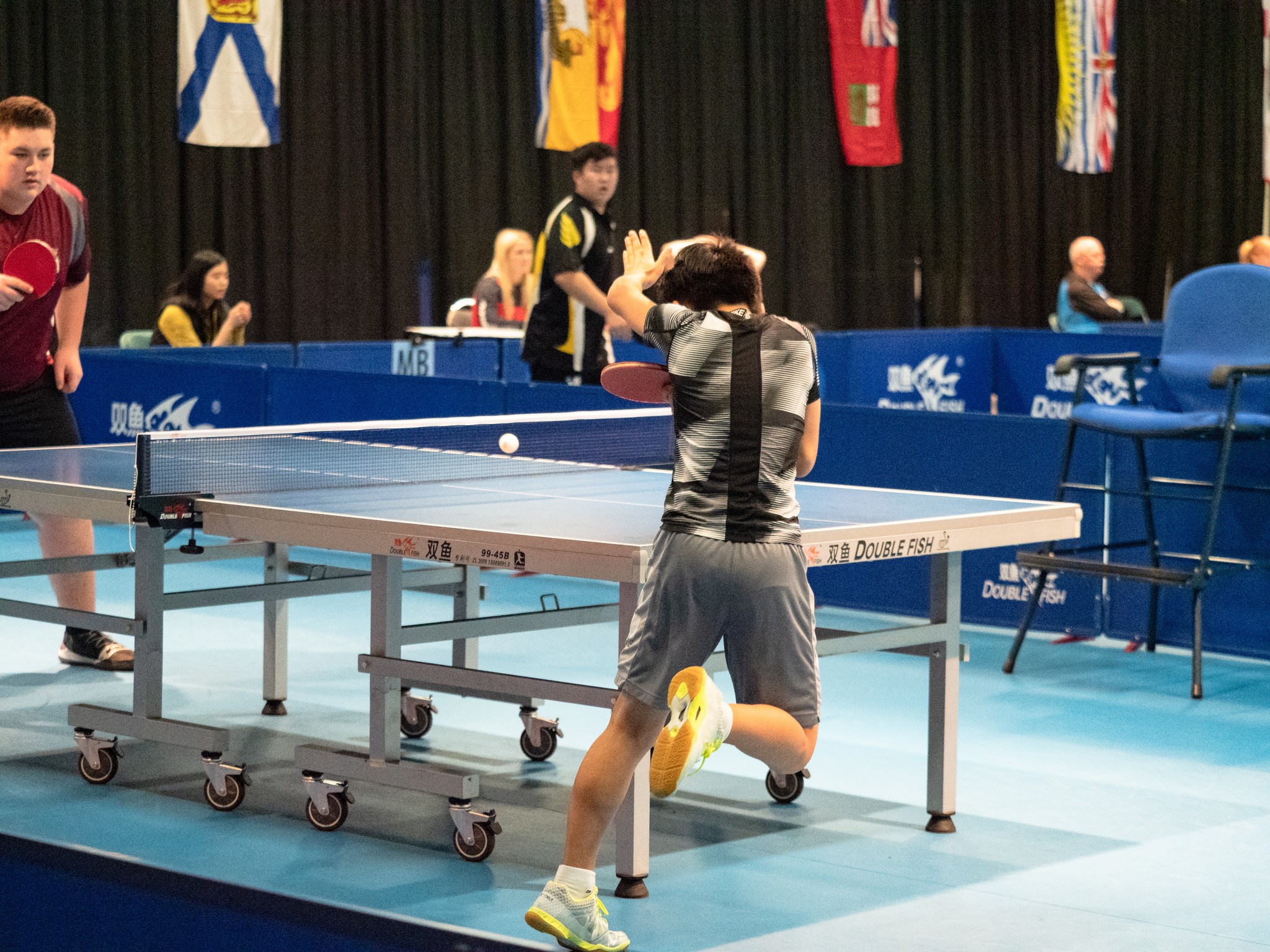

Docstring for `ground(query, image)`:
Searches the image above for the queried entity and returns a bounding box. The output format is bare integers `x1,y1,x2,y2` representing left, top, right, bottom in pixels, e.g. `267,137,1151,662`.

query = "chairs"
446,297,476,328
1113,296,1149,325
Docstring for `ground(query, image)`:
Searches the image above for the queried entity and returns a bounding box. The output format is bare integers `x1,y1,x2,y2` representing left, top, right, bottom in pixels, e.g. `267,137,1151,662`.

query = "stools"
1000,264,1270,700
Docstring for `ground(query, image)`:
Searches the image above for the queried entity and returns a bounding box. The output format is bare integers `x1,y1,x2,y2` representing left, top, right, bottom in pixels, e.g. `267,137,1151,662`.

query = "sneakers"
525,881,630,952
58,629,137,671
648,667,723,797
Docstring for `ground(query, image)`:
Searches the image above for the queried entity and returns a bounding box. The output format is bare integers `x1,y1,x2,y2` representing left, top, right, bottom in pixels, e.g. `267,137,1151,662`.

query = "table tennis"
499,433,520,455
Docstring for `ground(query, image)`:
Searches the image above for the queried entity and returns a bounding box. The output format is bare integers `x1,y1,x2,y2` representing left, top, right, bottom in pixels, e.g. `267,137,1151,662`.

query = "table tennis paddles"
2,239,60,303
600,361,674,404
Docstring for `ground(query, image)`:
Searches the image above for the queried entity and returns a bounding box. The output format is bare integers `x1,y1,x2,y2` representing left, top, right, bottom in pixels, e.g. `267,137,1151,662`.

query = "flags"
174,0,282,148
532,0,627,150
827,0,902,166
1054,0,1119,173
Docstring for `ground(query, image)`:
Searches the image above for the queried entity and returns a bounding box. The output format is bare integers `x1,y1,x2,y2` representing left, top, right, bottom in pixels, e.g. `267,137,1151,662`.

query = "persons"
1056,236,1124,337
1239,236,1270,268
0,96,134,671
472,228,534,329
523,228,820,952
151,249,253,347
519,142,633,385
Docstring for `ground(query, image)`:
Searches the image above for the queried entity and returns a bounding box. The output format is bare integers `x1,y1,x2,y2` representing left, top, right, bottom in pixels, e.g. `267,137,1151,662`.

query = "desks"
405,323,527,339
0,404,1081,900
